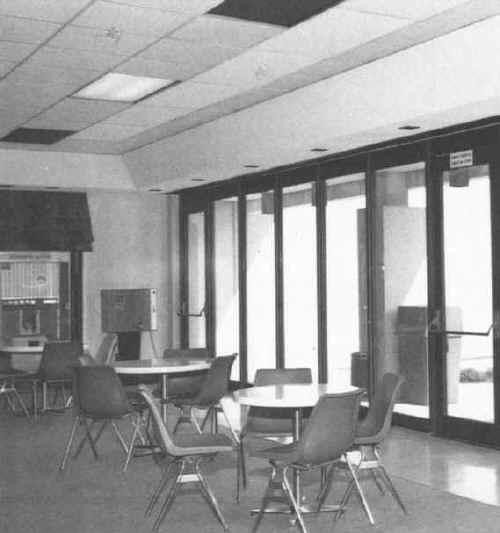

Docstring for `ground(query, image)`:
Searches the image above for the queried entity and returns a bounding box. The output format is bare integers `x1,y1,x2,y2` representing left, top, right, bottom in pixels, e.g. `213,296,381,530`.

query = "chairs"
166,352,240,460
161,346,214,440
312,368,407,523
251,388,377,529
74,351,146,454
134,389,235,531
3,333,95,426
238,365,315,490
57,362,159,476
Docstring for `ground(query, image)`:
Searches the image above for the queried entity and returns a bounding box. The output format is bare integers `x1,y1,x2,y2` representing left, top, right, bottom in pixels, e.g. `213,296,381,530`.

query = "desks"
232,381,364,516
103,361,210,456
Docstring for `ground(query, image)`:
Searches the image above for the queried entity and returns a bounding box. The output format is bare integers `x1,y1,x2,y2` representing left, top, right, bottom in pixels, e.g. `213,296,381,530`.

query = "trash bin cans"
350,352,369,387
